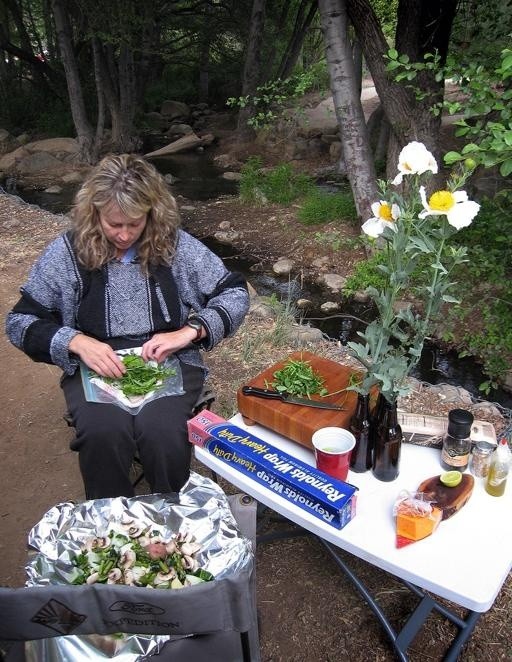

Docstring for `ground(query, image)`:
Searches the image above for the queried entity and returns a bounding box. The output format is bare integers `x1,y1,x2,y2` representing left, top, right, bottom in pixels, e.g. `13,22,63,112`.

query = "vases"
372,399,404,481
347,393,377,473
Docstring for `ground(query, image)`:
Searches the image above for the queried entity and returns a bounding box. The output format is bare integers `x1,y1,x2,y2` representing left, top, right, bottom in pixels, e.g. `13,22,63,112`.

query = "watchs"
183,320,202,343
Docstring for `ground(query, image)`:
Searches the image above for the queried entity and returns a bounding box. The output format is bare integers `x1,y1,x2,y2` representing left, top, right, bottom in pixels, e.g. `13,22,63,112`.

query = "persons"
5,153,251,497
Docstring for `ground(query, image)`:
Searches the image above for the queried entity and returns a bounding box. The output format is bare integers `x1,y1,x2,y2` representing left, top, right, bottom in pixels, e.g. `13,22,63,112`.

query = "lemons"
440,471,462,488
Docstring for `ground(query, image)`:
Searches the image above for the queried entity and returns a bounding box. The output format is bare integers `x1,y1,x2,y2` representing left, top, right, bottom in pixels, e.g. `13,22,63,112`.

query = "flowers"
353,139,438,397
392,188,483,393
68,526,213,592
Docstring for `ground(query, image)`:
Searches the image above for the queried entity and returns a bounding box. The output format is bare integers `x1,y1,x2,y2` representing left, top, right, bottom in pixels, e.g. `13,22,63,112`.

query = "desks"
189,400,510,660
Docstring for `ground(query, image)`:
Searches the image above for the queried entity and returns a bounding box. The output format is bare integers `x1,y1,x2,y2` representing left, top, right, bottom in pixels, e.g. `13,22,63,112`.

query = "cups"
438,409,475,469
312,426,357,483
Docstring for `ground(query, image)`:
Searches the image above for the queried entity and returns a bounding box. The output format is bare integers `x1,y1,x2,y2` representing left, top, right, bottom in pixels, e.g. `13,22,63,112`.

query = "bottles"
348,382,401,481
484,435,511,497
469,440,491,477
